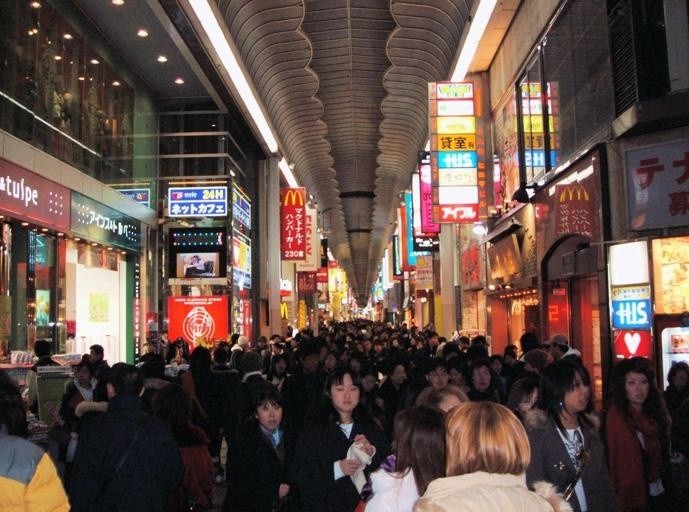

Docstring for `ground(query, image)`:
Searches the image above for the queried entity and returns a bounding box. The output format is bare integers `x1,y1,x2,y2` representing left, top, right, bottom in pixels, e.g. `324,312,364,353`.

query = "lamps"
512,181,537,203
574,239,630,254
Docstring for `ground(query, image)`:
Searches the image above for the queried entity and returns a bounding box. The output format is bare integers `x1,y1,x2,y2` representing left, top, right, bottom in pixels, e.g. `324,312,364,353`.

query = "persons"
184,253,205,277
0,318,688,512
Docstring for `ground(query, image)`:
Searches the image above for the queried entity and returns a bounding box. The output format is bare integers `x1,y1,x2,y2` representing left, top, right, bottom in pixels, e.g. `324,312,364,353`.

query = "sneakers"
215,475,224,483
